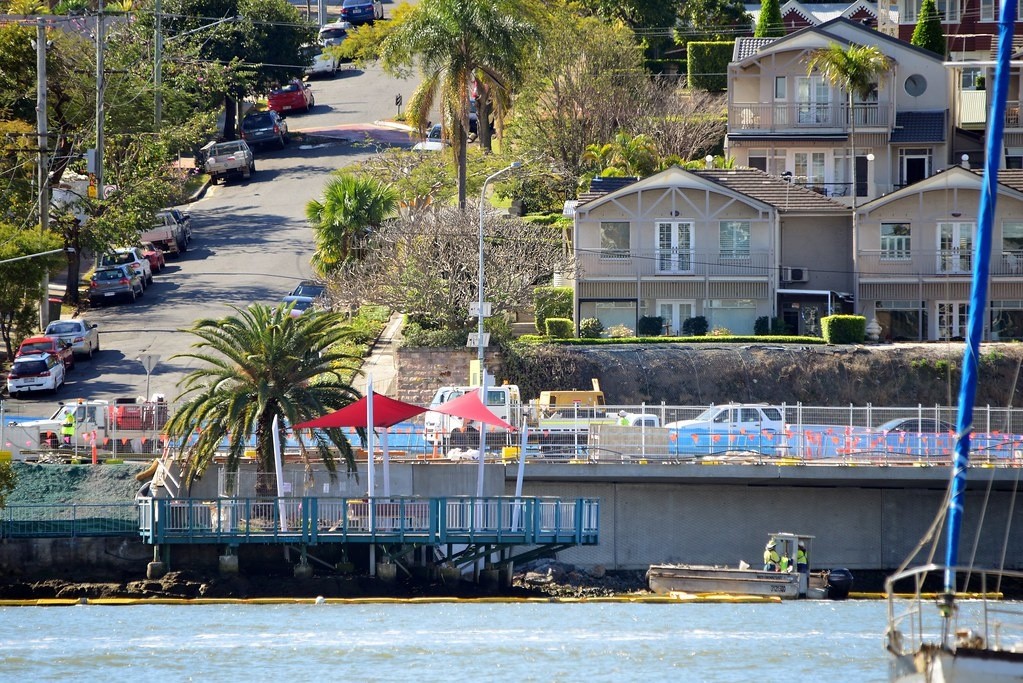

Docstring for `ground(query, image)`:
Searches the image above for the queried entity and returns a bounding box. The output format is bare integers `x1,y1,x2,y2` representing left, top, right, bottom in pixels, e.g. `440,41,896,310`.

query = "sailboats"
881,0,1023,683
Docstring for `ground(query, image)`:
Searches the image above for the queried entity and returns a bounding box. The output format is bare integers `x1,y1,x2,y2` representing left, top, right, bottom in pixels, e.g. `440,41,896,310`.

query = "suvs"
665,402,786,431
98,246,154,290
159,209,193,242
133,212,188,259
7,351,65,399
14,334,75,372
239,108,288,150
44,319,99,360
315,21,357,49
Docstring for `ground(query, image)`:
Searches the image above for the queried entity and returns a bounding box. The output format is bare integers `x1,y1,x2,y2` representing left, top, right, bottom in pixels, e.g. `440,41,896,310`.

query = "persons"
618,409,629,426
796,541,808,573
763,540,780,572
59,410,75,444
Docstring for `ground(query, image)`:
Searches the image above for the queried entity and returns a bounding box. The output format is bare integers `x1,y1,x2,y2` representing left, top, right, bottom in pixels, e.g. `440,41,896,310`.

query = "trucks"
421,382,619,457
203,140,256,186
6,399,180,455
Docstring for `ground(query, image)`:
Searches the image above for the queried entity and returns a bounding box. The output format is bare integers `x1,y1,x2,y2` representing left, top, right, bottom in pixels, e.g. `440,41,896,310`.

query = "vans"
467,99,495,132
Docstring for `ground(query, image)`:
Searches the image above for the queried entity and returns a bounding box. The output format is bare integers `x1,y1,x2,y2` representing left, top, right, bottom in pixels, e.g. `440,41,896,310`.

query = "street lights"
479,161,523,384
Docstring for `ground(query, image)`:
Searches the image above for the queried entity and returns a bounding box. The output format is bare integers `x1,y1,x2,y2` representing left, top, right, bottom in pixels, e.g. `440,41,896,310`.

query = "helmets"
66,408,71,413
619,410,627,416
767,540,777,549
798,540,804,547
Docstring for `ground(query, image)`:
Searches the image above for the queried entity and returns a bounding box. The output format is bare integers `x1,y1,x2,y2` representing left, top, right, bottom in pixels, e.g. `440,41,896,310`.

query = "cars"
339,0,384,27
870,416,958,434
271,278,331,328
428,124,453,146
90,264,143,307
131,241,166,274
404,142,450,176
300,38,343,78
267,79,315,114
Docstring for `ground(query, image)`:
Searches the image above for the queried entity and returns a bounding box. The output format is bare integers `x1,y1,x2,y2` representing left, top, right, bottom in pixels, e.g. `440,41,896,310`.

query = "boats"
644,530,853,602
